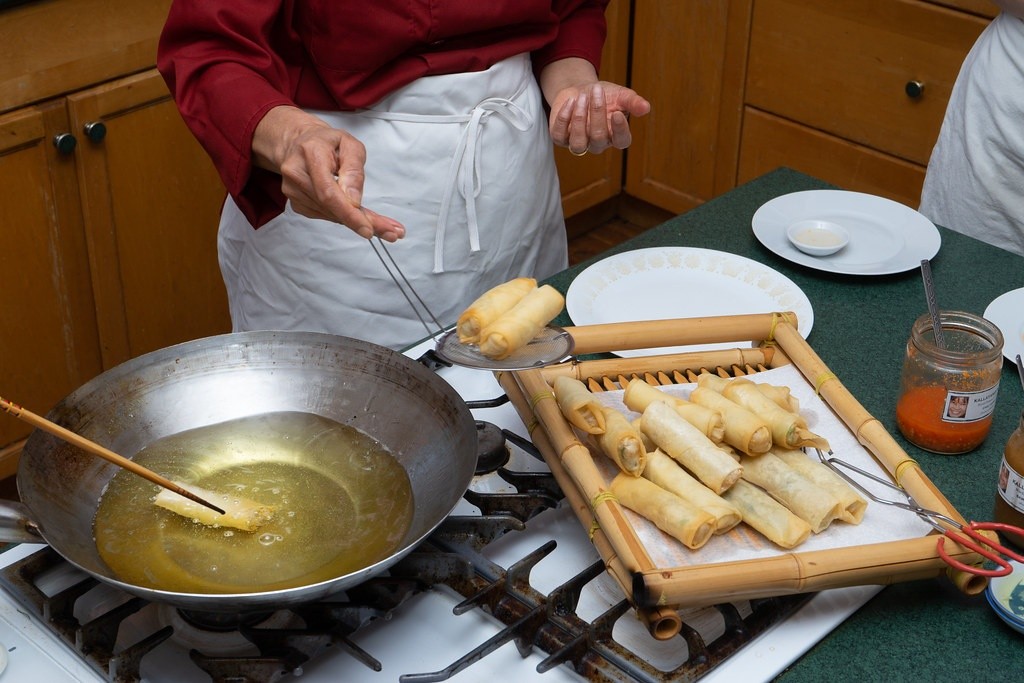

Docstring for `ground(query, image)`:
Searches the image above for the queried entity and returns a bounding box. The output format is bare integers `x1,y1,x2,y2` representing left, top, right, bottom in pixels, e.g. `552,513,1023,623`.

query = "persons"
154,0,652,353
918,0,1024,256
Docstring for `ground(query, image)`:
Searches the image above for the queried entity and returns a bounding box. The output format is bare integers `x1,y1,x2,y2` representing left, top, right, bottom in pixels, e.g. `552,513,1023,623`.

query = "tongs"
801,446,1024,577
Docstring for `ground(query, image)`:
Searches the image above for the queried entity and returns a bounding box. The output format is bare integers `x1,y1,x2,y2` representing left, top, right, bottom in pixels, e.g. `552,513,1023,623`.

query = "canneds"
896,310,1005,454
993,407,1024,553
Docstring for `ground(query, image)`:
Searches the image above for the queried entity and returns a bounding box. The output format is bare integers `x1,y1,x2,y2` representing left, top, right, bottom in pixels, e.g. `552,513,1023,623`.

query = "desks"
539,165,1024,683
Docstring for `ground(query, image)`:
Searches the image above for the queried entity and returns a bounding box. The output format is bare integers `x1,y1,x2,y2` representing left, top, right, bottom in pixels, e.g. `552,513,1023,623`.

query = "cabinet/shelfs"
713,0,1002,210
0,68,232,484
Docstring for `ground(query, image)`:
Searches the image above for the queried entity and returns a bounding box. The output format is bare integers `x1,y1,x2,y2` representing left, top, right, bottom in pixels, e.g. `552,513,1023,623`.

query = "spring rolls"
553,373,868,548
456,277,564,360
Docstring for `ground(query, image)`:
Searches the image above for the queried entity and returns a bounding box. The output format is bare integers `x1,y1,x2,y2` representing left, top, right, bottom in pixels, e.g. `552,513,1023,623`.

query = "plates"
985,559,1024,632
751,190,942,275
566,247,814,358
788,220,850,256
983,286,1024,369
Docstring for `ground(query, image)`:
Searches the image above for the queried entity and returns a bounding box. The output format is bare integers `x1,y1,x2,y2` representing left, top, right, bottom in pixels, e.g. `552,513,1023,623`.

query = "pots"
0,329,480,606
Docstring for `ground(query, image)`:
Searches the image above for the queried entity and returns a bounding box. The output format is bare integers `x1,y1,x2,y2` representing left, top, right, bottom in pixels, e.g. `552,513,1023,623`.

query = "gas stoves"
0,349,882,683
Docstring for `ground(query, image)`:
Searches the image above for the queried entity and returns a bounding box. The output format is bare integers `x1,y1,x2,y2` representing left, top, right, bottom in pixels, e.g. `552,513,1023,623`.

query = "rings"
567,145,591,158
